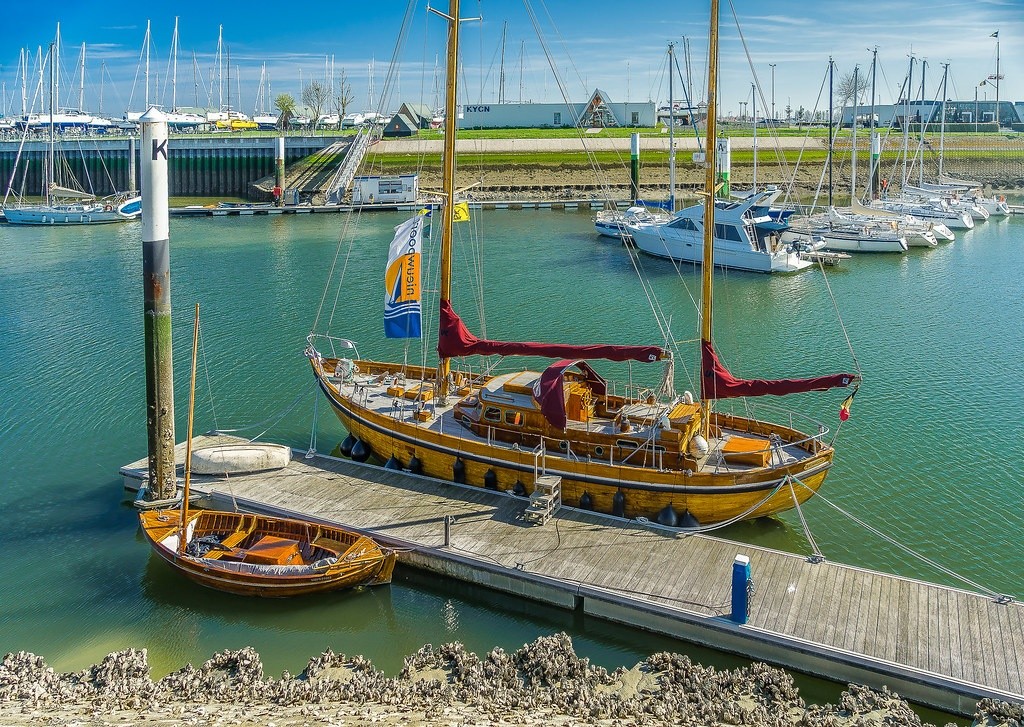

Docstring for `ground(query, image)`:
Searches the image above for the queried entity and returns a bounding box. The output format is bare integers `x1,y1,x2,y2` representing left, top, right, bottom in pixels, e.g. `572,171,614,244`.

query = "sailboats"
305,0,867,527
0,15,449,224
132,301,400,597
596,38,1016,275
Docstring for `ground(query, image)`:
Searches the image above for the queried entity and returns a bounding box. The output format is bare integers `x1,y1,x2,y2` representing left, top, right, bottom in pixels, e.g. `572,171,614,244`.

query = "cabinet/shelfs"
524,474,562,526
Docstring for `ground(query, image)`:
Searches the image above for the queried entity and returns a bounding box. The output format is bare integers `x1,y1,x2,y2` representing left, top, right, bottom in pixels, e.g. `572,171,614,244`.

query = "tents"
294,102,433,138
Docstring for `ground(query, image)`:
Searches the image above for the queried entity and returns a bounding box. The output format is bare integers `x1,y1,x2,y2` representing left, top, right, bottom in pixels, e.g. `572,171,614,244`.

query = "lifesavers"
104,204,114,212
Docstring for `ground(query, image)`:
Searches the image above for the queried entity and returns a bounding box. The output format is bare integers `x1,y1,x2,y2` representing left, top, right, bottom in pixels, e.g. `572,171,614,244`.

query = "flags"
989,30,999,38
980,73,1004,87
384,200,471,340
